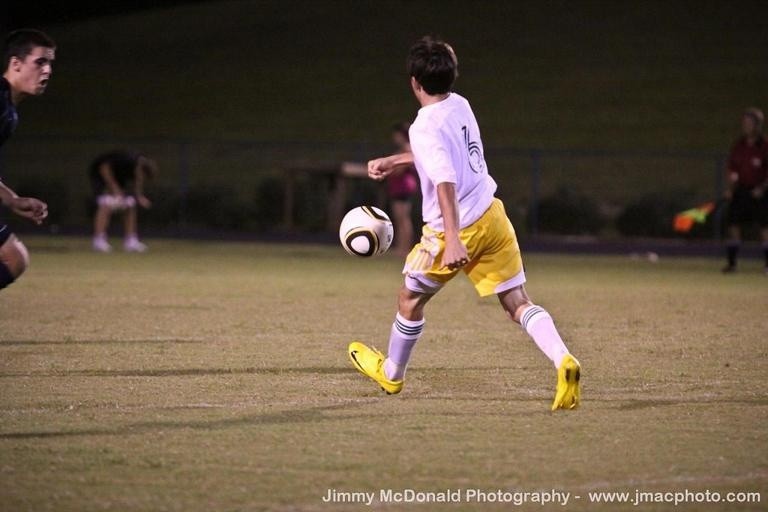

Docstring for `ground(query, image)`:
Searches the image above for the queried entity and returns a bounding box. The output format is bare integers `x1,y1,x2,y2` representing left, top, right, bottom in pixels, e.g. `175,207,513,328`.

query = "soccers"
340,206,394,257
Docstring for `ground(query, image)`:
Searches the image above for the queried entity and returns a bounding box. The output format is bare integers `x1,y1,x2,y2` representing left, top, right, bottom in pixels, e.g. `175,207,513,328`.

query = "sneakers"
551,353,581,413
91,233,149,253
348,341,406,397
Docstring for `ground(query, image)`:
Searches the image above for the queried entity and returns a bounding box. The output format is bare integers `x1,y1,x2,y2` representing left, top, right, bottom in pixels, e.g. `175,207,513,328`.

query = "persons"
85,139,153,254
719,108,768,274
383,122,418,263
348,34,582,413
1,25,57,293
